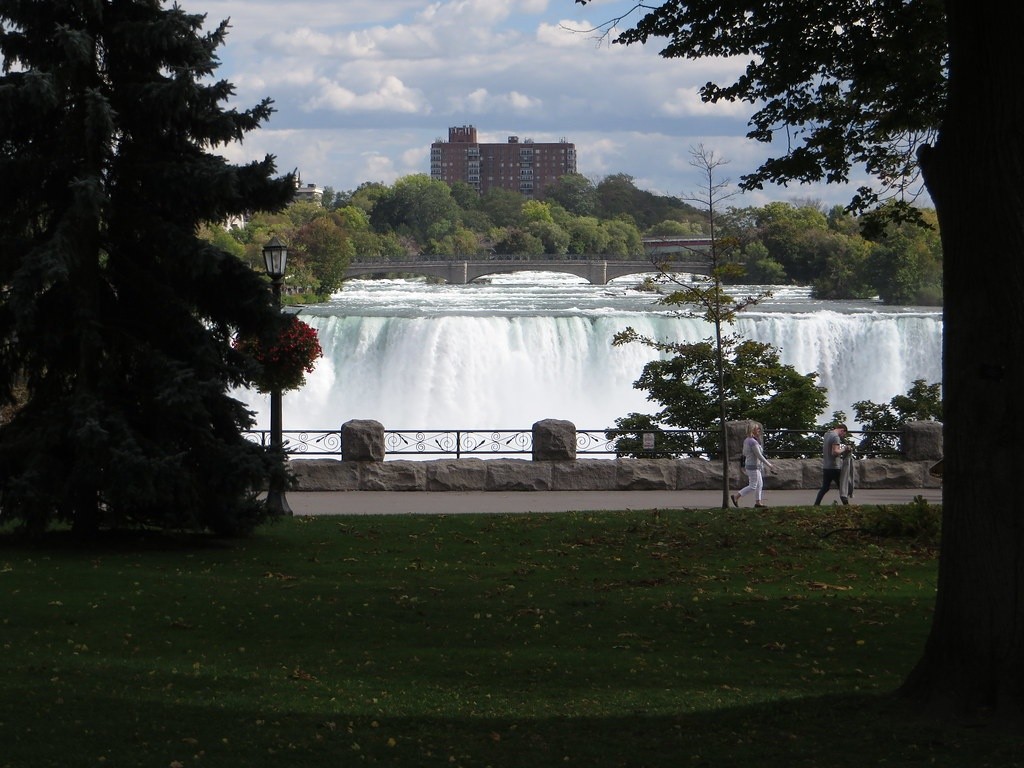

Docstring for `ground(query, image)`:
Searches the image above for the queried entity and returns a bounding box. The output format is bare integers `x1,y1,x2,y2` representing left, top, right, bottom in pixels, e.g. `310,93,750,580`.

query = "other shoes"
730,494,739,508
754,503,767,507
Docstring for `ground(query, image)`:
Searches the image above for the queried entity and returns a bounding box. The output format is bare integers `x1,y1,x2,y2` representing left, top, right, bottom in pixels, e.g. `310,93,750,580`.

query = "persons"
728,424,774,508
814,424,851,506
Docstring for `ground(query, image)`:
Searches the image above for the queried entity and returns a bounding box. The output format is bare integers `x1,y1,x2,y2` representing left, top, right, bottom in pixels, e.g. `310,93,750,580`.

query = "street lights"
261,236,294,517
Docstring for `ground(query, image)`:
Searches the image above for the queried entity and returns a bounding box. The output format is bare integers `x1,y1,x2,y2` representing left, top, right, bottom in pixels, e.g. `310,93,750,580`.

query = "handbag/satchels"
740,454,746,467
